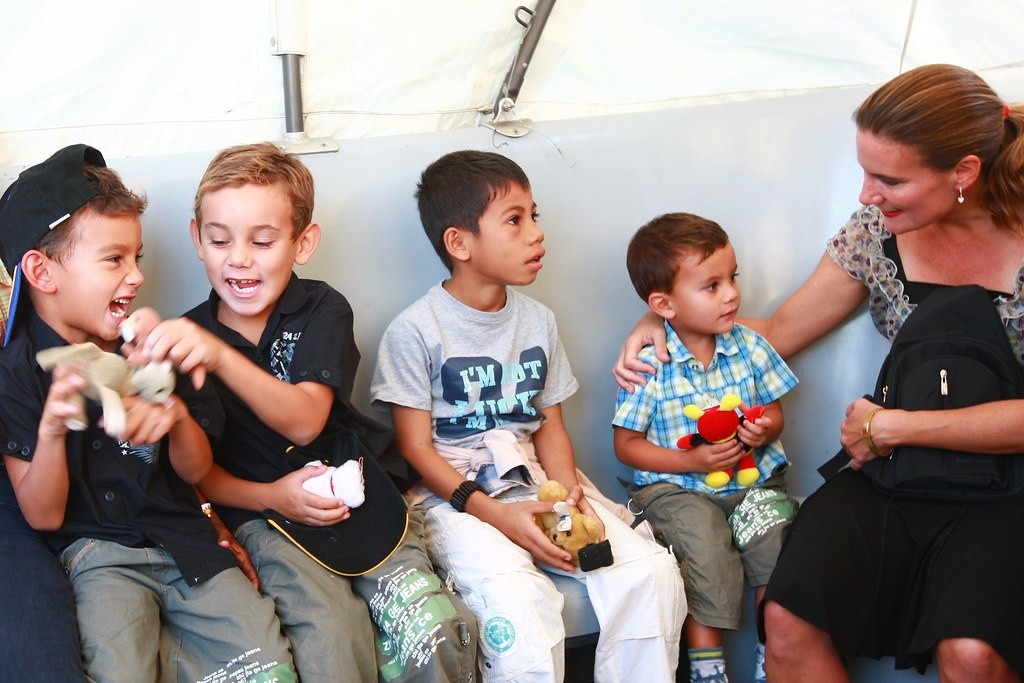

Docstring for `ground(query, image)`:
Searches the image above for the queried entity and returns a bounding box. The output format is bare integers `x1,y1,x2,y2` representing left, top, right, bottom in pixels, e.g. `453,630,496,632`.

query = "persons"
3,146,298,680
369,149,689,683
616,212,801,682
143,143,477,683
610,64,1024,683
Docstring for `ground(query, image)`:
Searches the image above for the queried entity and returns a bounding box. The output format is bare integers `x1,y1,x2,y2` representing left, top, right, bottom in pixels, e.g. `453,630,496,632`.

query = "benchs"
0,63,1023,683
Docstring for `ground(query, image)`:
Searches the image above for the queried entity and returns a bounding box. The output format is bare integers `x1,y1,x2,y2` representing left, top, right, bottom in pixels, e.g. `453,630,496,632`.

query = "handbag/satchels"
855,283,1024,510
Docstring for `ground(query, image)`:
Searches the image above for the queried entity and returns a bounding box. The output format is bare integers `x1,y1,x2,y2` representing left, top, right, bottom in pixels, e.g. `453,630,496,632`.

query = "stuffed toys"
677,393,765,488
301,457,365,510
33,341,176,436
535,480,614,572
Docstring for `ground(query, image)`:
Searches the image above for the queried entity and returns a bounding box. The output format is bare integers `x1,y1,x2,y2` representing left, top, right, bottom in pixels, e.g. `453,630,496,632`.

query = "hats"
0,143,107,347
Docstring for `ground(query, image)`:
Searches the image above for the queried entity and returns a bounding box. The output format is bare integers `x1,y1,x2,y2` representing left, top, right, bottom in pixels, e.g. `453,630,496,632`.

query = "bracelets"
862,408,893,457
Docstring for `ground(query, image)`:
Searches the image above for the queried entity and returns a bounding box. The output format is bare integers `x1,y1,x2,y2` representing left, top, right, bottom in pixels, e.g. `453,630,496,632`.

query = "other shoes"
753,642,766,683
688,661,729,683
